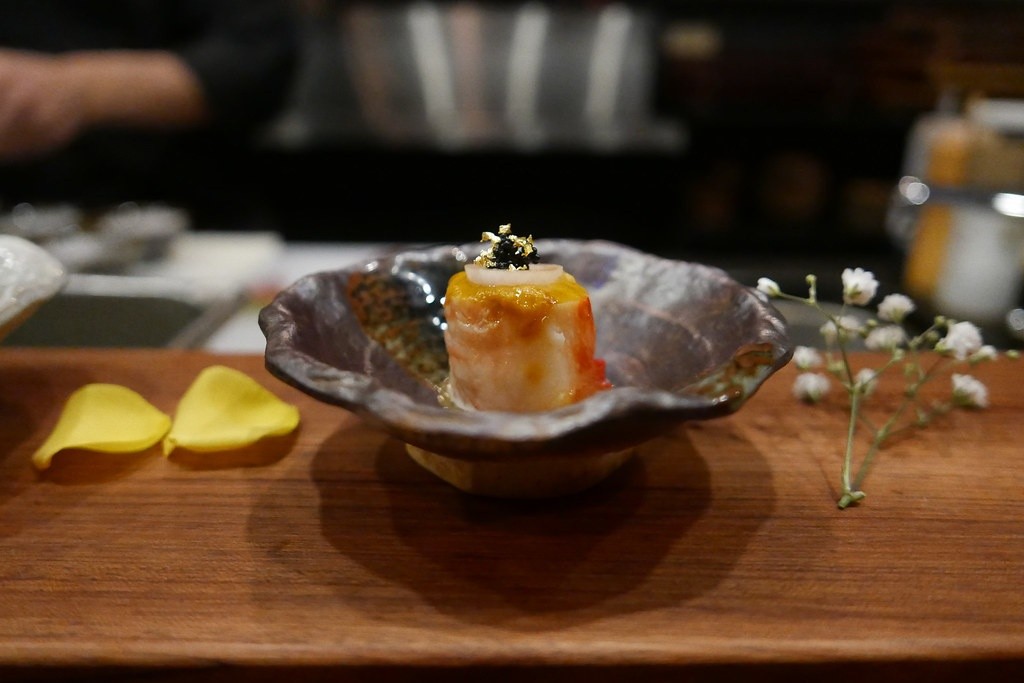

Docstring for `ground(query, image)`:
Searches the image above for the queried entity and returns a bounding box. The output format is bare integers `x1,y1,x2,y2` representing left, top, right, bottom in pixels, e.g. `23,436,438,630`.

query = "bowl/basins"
1,233,65,347
256,240,800,499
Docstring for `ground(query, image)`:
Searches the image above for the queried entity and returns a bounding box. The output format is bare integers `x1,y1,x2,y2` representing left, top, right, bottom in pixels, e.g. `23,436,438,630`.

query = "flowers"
754,265,999,510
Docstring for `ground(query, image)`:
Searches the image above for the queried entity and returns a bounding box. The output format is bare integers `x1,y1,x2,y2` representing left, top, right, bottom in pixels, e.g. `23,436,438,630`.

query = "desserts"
444,223,614,413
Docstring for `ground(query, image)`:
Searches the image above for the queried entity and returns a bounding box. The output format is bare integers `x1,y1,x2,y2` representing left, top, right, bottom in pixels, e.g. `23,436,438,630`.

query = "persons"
0,0,304,215
